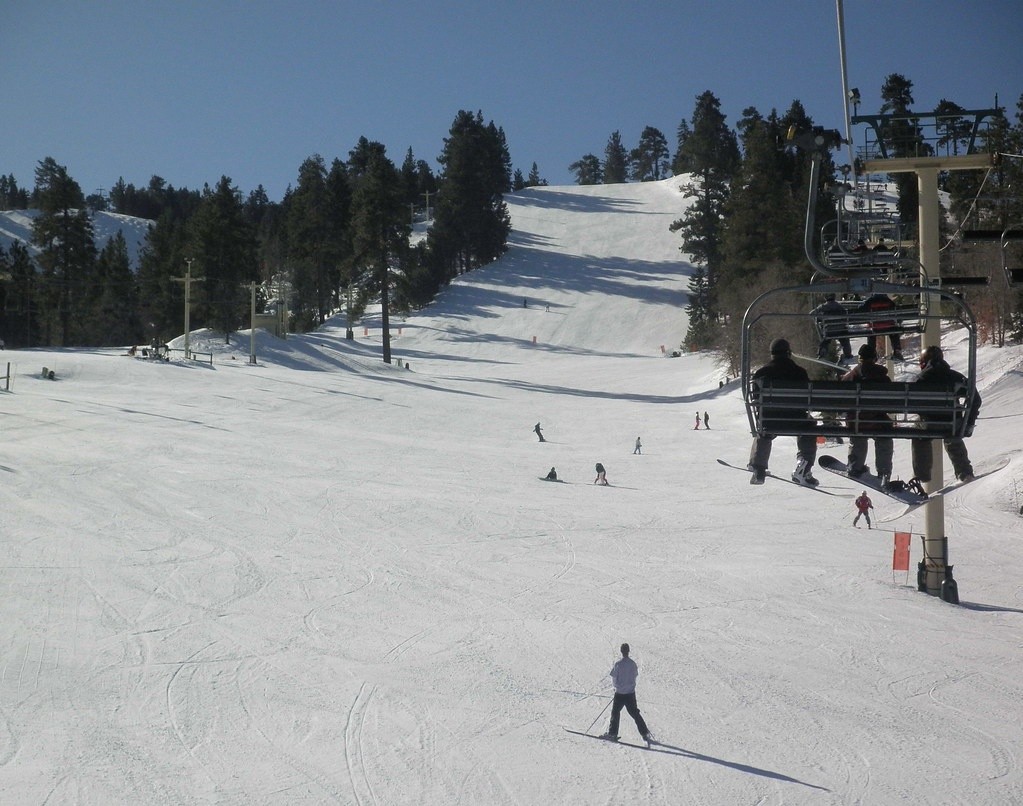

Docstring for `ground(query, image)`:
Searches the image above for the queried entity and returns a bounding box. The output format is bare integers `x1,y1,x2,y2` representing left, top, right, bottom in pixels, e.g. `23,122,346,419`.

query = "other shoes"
878,471,892,479
959,470,974,481
890,351,905,361
846,464,869,476
599,732,617,738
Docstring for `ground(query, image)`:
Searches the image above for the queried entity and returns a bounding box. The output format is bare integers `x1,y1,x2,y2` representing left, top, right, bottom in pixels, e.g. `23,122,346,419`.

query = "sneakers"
791,459,819,487
752,466,766,480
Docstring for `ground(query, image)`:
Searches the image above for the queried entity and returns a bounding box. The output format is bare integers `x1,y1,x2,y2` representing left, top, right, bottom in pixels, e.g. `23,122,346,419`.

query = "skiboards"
716,458,857,500
561,708,651,739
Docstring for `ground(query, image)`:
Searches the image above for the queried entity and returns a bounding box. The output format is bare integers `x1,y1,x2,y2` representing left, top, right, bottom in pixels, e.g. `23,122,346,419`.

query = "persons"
633,437,642,455
912,346,982,483
851,239,869,267
703,411,710,429
853,490,874,529
747,338,819,489
532,421,545,442
523,298,527,308
851,238,889,276
695,411,701,429
847,293,905,362
545,466,557,480
544,302,550,312
598,643,651,740
815,293,853,360
841,343,894,479
594,463,608,486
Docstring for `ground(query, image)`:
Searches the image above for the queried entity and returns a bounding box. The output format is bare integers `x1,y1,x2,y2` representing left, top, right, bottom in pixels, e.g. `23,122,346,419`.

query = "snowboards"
818,452,929,505
878,456,1012,524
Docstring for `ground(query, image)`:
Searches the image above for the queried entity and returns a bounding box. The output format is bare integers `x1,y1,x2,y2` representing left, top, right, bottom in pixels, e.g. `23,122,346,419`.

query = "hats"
861,490,866,494
858,344,877,360
824,293,836,300
770,338,791,353
